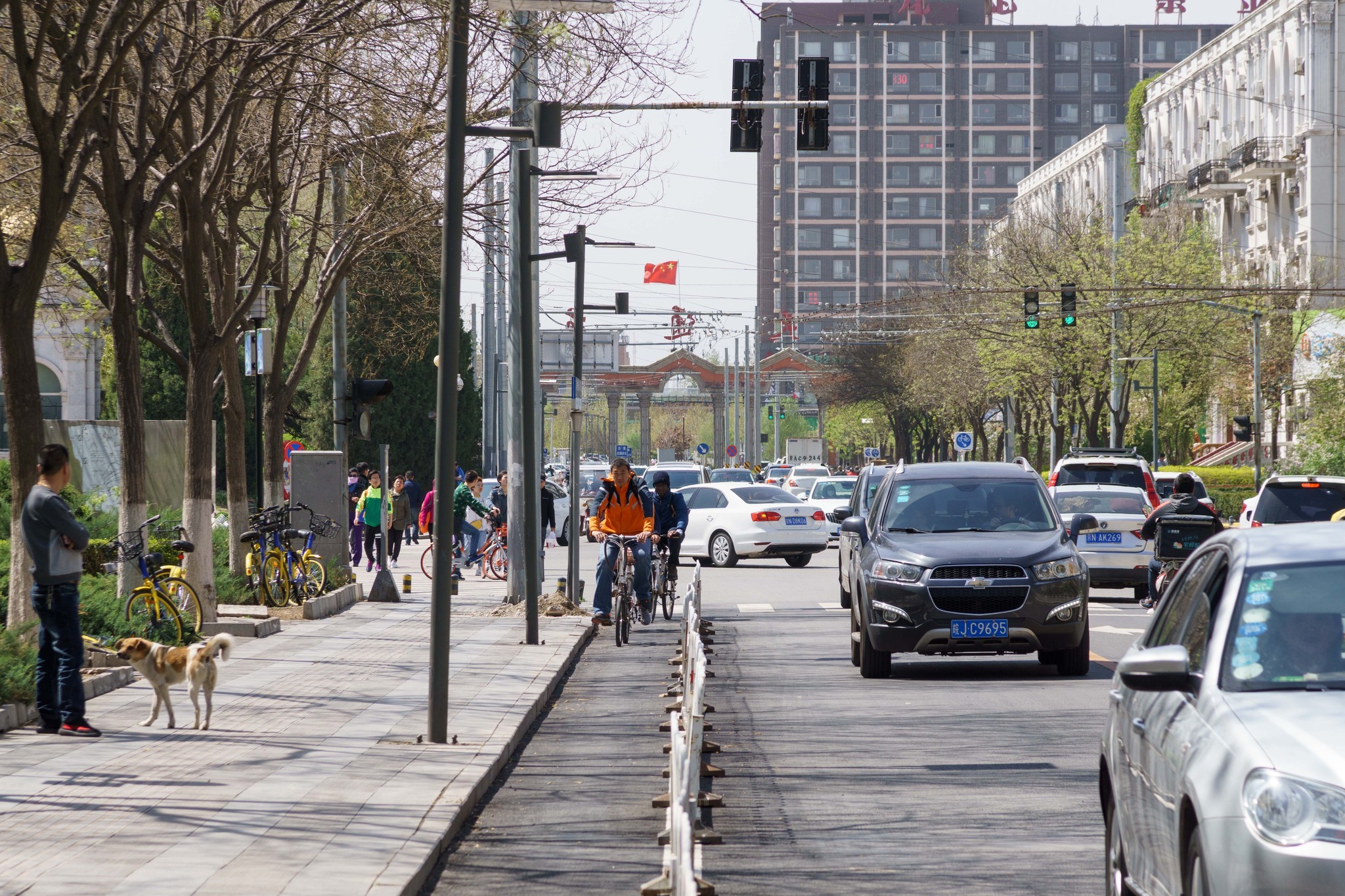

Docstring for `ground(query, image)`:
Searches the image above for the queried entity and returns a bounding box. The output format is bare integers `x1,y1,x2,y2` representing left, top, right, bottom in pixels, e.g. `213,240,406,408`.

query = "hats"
540,473,546,482
653,471,670,488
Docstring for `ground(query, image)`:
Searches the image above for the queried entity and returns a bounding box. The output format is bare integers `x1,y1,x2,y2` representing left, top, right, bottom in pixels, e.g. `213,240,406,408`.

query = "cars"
466,448,957,610
1047,482,1157,600
1097,522,1345,896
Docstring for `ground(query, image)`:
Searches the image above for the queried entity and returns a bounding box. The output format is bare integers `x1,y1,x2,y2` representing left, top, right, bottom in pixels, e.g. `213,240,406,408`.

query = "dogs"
115,632,233,731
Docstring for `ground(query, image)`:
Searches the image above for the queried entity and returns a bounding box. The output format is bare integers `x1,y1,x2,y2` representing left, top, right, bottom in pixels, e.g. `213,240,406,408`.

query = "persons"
1253,585,1345,684
1157,454,1169,468
1136,472,1224,611
979,491,1038,531
343,455,688,627
21,444,103,736
816,463,868,498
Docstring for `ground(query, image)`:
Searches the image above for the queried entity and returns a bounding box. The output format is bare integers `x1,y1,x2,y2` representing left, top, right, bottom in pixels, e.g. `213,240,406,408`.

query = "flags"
643,261,678,284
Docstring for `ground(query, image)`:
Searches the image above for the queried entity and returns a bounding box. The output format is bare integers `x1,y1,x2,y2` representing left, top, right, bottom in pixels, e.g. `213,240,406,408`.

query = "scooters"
1141,502,1225,610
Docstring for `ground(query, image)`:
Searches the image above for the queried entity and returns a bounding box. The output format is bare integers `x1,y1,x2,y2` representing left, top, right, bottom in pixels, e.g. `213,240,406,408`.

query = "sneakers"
1140,599,1154,609
366,558,382,572
387,555,398,568
639,607,652,625
468,554,485,565
592,613,611,624
667,566,675,581
453,572,465,581
475,567,483,576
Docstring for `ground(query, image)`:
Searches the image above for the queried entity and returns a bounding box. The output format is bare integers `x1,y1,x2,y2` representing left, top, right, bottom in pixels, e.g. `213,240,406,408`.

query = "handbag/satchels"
419,491,433,534
546,531,556,548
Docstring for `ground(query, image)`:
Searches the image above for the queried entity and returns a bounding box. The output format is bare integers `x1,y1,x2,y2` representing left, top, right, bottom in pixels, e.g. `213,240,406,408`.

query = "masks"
348,477,358,483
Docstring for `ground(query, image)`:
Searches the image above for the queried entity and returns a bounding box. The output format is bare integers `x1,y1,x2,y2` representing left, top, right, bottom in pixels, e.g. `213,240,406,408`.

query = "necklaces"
36,482,49,490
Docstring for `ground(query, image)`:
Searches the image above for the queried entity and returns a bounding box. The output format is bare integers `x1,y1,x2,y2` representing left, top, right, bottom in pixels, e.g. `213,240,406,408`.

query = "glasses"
349,474,357,478
365,467,380,480
654,484,668,488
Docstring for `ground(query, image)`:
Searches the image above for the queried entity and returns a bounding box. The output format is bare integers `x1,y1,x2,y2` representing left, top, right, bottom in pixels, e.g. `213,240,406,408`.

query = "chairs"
1068,472,1086,482
823,485,837,497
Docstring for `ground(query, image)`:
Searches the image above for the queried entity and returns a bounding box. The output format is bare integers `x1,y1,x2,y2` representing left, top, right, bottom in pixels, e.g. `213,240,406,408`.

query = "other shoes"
36,720,62,734
349,549,358,566
405,538,419,546
58,719,103,737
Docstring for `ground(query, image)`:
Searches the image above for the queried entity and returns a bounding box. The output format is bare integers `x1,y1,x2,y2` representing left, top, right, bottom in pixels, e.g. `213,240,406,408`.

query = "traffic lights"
768,405,785,420
1024,285,1040,329
352,378,394,404
1233,415,1251,442
1061,283,1077,327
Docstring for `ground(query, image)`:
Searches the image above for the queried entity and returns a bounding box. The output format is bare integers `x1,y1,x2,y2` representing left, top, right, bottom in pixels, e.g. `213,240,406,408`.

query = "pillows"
1110,497,1142,514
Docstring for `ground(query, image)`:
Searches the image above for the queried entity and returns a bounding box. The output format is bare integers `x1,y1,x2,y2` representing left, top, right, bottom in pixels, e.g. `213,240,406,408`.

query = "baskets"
247,507,292,547
309,514,343,538
493,522,508,537
97,530,142,563
153,520,182,545
215,515,229,528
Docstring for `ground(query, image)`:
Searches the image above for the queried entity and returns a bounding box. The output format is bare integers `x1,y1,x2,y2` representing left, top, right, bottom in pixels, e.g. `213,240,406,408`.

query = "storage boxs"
1151,515,1216,558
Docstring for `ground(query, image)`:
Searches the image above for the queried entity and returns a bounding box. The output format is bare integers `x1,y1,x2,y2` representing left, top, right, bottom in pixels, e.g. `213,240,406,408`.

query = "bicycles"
99,513,206,647
600,532,640,647
480,509,507,580
239,498,343,608
420,512,507,580
650,533,682,622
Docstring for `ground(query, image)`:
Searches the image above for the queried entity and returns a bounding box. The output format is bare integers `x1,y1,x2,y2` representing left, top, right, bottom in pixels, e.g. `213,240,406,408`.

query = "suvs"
840,456,1099,678
1046,445,1173,508
1238,472,1345,528
1152,470,1216,511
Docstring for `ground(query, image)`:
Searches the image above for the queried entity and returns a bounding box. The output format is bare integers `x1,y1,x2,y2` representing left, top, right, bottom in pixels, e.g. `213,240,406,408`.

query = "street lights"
238,284,283,521
526,160,624,646
1200,298,1262,493
428,118,539,745
564,226,658,609
1117,348,1159,472
